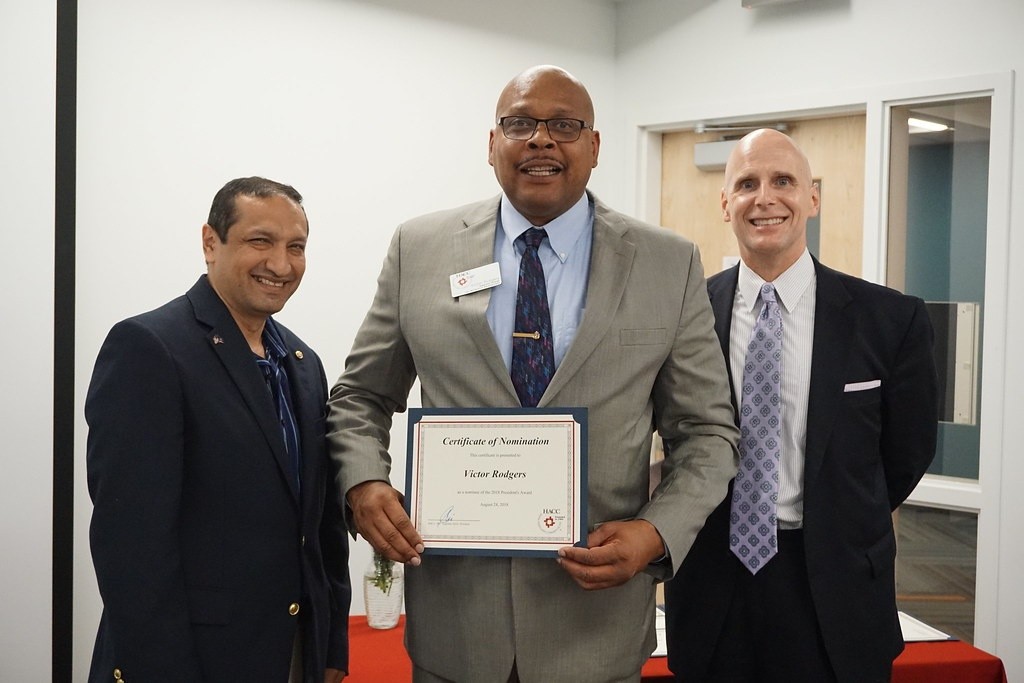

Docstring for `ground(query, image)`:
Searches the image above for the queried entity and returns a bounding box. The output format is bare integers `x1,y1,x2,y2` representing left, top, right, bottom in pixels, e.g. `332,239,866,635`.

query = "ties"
511,228,556,408
730,282,784,576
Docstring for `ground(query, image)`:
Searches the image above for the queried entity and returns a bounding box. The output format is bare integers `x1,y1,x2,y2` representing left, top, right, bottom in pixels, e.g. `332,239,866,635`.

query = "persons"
326,66,744,683
83,175,350,683
656,127,940,683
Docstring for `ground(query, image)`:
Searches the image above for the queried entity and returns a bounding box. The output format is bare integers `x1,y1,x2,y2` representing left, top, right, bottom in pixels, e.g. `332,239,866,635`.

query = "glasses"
492,116,595,143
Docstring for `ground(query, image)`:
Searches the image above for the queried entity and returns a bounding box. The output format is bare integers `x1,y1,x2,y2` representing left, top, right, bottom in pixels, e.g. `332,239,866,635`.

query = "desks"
340,603,1007,683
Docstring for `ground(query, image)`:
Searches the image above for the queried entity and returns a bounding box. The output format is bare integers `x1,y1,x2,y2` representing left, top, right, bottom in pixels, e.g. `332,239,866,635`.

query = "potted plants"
364,552,403,629
